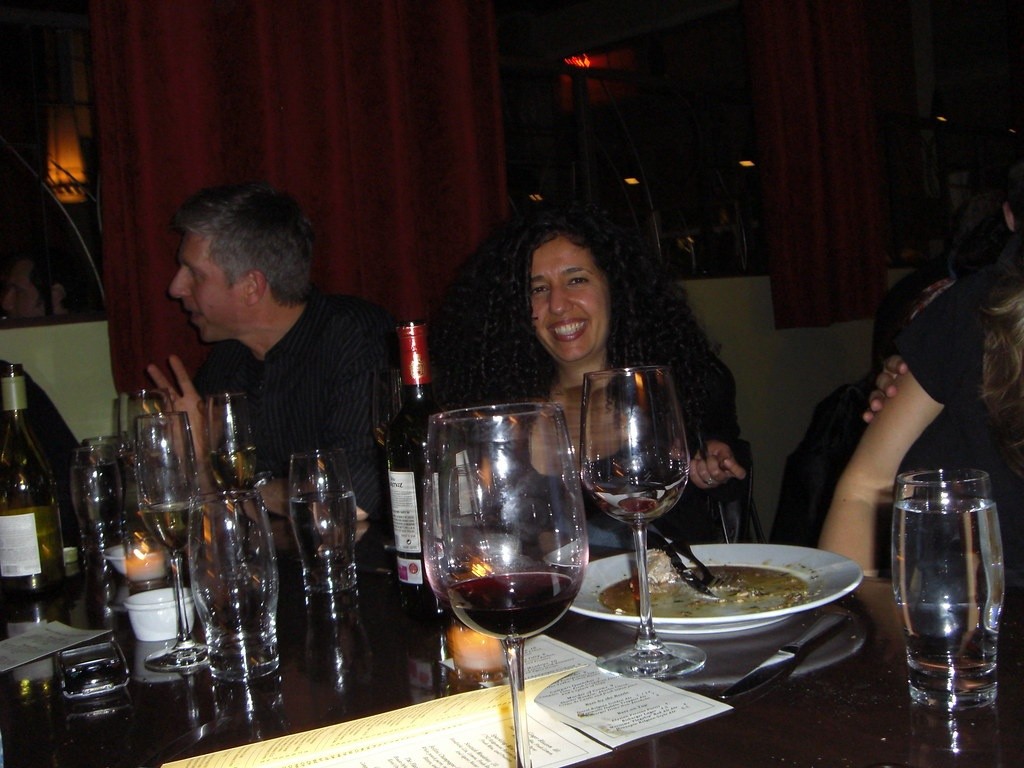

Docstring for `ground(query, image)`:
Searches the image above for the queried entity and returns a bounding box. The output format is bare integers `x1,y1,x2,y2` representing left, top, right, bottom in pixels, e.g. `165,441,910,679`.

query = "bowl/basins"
103,541,171,581
123,585,196,641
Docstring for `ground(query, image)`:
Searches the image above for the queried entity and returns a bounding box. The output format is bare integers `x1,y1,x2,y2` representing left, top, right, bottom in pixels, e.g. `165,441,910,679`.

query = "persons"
0,152,1024,588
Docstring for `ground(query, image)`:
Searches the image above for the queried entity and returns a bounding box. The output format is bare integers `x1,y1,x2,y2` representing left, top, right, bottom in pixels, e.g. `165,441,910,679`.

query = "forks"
676,540,731,588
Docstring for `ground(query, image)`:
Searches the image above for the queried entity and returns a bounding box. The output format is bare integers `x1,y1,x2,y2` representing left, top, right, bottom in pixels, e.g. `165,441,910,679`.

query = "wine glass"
135,410,215,673
434,401,590,768
207,391,267,580
579,365,706,679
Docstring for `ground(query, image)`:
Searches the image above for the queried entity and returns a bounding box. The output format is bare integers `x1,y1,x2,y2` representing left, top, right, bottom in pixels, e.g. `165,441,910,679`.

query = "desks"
0,549,1024,768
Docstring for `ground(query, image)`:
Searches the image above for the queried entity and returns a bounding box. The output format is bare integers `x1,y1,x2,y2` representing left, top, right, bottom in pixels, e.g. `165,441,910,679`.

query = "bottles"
0,363,65,591
383,318,464,620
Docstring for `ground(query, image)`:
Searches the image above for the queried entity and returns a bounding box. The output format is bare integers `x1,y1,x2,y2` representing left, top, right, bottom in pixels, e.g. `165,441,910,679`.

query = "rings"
704,477,712,485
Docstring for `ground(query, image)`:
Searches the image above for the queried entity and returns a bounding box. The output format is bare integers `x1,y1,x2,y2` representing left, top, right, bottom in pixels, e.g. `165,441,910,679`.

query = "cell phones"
54,639,130,699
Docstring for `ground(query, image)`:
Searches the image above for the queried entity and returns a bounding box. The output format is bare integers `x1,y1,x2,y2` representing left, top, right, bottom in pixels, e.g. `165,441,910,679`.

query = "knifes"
719,611,848,698
645,529,718,599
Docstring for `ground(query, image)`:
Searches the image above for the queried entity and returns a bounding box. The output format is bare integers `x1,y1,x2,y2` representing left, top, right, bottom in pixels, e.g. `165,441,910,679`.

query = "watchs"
248,470,276,490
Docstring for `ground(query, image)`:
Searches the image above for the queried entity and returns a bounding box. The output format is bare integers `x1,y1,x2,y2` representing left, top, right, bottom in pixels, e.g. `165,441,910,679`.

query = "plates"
565,543,864,640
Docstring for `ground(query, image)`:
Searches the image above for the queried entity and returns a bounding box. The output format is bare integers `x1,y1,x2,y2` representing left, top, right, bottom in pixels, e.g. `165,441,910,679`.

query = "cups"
187,489,279,682
79,436,126,467
422,415,522,620
288,448,358,594
890,468,1007,711
71,444,125,551
119,387,182,590
371,367,415,450
